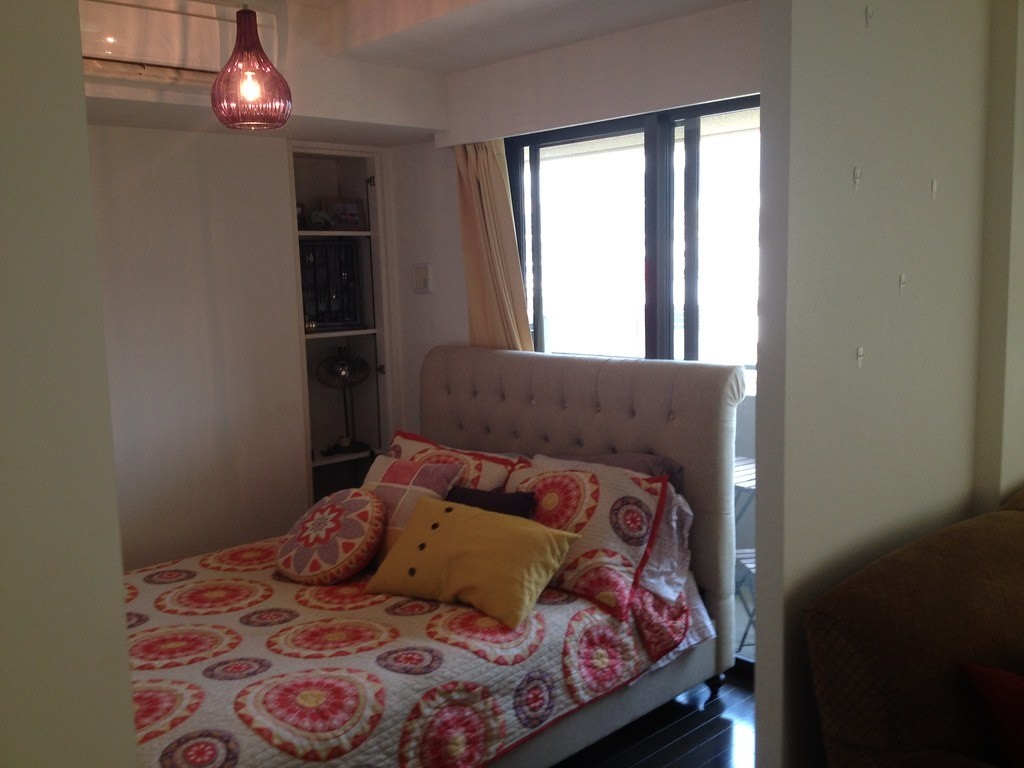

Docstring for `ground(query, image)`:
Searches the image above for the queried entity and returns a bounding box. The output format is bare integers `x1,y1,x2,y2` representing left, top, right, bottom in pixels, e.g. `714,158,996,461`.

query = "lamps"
210,4,292,132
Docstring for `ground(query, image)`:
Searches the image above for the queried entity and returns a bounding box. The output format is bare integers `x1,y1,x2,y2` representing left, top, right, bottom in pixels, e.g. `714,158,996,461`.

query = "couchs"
801,488,1024,768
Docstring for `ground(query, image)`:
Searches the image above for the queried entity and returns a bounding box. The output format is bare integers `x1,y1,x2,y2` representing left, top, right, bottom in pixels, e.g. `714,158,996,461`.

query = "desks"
732,454,756,523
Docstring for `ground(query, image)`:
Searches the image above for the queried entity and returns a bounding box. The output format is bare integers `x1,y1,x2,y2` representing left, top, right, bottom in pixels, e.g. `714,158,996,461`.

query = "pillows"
360,453,467,574
275,486,377,586
362,494,582,631
386,431,511,492
501,453,667,624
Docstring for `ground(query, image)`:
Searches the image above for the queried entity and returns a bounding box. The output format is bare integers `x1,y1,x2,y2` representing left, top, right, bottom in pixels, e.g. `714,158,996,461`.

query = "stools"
734,548,757,653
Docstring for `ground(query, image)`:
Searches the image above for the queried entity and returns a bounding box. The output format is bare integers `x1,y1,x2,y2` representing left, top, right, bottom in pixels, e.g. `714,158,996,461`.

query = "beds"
122,346,747,768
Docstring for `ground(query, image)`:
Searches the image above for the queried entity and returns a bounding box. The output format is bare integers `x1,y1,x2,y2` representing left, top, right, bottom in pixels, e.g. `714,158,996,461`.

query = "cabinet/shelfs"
285,140,391,513
